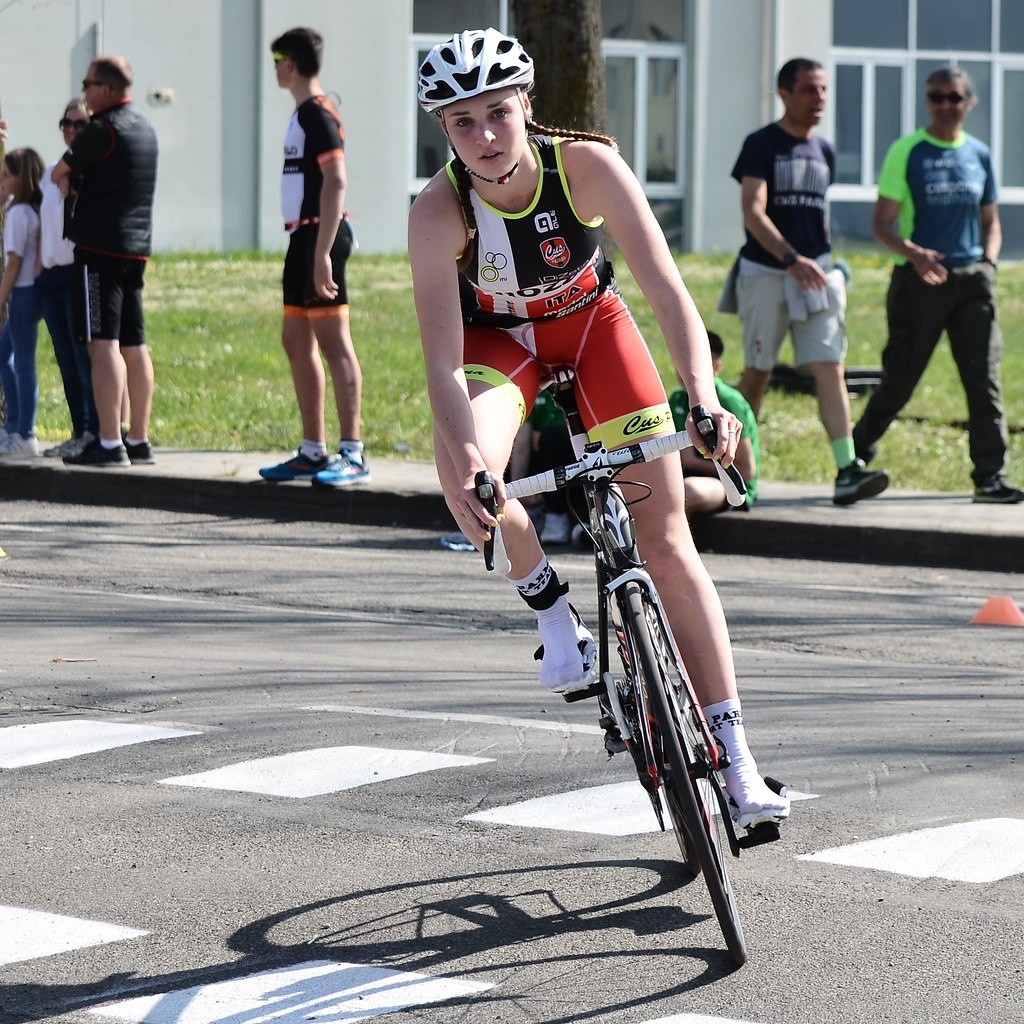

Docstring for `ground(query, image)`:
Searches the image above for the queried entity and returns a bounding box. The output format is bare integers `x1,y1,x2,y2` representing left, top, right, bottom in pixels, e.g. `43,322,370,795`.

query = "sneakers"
0,427,38,456
259,448,330,481
540,513,571,541
970,478,1024,504
830,461,888,507
313,449,370,486
43,423,154,468
511,556,586,690
701,699,790,826
439,532,475,552
573,524,600,545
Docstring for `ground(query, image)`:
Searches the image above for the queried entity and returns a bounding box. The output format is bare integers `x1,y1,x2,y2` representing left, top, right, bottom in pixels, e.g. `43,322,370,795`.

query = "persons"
259,27,370,490
407,25,791,829
0,99,130,459
51,56,158,468
440,328,758,549
851,68,1024,503
718,58,891,507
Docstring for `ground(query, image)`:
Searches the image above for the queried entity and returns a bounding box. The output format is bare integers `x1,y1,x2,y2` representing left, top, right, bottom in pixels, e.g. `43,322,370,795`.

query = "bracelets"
784,249,798,269
978,256,997,270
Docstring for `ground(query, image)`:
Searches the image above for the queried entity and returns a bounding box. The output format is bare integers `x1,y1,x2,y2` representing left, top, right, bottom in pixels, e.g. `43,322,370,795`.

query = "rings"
729,429,736,434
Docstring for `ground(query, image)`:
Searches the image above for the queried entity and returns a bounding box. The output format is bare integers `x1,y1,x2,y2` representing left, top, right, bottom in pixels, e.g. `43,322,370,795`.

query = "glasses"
270,52,287,62
925,89,969,105
83,79,103,90
59,118,88,134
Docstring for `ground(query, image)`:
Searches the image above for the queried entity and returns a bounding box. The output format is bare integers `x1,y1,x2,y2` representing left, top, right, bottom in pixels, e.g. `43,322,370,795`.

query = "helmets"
416,26,537,114
